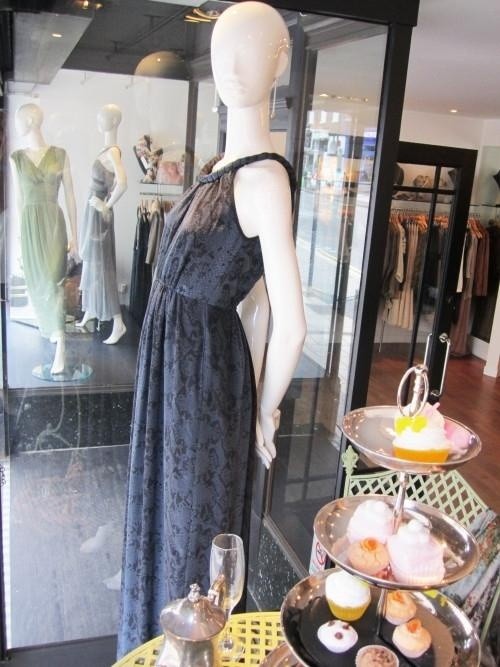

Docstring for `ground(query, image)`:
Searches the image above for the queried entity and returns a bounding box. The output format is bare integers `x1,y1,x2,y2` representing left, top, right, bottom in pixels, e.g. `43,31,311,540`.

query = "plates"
311,495,481,592
276,567,481,667
338,405,483,475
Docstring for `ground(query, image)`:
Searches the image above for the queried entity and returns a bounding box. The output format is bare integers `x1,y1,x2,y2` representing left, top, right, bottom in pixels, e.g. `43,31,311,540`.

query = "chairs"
339,443,500,648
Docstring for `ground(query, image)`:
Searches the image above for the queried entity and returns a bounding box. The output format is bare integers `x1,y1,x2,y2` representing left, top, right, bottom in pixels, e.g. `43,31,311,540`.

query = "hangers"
377,205,493,238
134,192,179,218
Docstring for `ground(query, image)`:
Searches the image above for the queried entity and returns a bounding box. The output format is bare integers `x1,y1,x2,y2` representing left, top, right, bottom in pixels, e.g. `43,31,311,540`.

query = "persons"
118,0,304,657
75,103,127,345
10,102,79,373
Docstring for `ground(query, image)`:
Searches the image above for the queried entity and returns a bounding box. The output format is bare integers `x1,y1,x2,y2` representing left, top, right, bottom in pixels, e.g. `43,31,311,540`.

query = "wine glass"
211,534,244,661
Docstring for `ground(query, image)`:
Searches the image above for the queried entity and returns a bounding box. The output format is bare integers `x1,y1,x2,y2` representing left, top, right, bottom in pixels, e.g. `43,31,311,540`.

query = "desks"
96,607,470,666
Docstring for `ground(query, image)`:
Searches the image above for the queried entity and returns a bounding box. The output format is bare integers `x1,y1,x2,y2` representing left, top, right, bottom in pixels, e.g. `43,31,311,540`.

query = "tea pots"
149,572,228,667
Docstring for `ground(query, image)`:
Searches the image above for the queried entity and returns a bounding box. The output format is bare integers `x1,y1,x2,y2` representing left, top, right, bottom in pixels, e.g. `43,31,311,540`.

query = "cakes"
316,398,449,667
